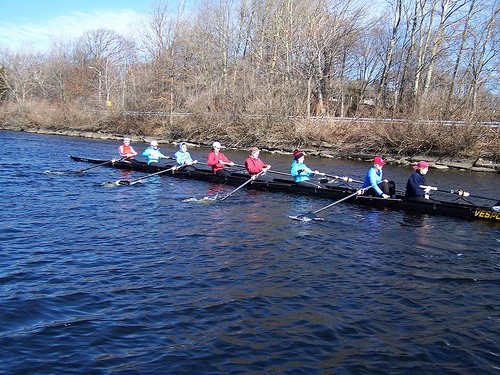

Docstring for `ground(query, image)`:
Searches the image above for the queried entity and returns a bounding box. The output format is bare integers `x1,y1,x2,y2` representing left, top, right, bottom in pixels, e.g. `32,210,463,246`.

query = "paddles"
168,157,207,165
312,187,372,215
221,169,267,199
79,156,122,171
122,163,186,186
306,170,363,183
235,163,291,175
432,188,500,202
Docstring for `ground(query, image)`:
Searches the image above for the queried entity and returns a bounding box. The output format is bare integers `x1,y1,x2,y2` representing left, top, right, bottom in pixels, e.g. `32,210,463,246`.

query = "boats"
67,154,500,223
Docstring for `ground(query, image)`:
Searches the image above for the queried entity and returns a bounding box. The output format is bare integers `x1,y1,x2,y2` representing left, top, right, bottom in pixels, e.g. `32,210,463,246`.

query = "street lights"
89,66,101,110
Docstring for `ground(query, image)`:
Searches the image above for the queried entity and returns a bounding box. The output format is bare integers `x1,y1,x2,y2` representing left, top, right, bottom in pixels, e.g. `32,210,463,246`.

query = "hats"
211,142,226,149
149,141,158,146
372,157,385,166
412,161,429,170
294,151,303,160
249,147,261,152
122,138,130,144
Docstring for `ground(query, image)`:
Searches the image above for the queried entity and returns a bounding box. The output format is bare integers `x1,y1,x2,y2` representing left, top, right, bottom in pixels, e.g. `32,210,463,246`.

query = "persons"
142,141,170,165
404,160,432,201
245,147,273,181
171,142,198,171
208,141,235,176
289,149,327,189
118,138,138,159
362,157,396,198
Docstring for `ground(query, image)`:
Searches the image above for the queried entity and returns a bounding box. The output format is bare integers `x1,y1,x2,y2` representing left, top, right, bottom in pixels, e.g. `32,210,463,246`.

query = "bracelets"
381,193,384,196
